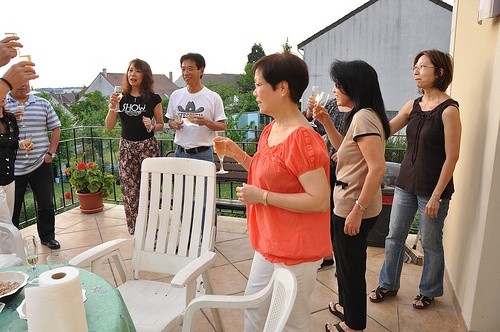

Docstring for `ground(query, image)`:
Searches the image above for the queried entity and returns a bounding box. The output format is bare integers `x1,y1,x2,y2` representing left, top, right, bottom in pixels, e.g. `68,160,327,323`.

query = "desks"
0,263,136,332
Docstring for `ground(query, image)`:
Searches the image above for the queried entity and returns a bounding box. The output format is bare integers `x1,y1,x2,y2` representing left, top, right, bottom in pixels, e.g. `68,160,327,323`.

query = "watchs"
47,152,55,158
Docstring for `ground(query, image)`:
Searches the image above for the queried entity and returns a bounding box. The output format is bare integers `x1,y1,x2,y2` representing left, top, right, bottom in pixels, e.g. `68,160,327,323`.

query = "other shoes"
318,259,334,270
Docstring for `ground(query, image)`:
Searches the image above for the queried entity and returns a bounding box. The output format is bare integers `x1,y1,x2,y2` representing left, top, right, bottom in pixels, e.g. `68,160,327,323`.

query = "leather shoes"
41,239,60,249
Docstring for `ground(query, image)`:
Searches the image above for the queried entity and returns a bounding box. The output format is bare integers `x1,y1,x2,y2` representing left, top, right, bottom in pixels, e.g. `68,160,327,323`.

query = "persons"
302,85,354,276
164,52,228,253
0,36,23,254
4,81,62,249
106,59,164,248
0,61,39,103
312,58,390,332
369,50,462,310
212,51,333,332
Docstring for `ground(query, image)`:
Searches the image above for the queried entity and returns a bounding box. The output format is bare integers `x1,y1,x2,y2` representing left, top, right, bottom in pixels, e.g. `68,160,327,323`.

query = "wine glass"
18,102,27,128
309,92,329,127
19,51,39,95
115,85,123,112
214,130,229,174
23,235,40,285
23,136,32,158
173,107,184,132
309,86,320,114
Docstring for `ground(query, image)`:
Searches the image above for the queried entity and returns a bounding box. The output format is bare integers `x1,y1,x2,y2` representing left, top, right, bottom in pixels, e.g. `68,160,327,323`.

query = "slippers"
329,302,345,322
325,322,364,332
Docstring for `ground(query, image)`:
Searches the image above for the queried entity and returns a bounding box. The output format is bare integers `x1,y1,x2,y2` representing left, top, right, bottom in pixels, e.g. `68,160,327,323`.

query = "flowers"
63,153,117,199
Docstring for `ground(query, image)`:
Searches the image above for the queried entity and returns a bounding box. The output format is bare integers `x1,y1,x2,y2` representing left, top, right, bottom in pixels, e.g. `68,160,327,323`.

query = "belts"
177,145,210,155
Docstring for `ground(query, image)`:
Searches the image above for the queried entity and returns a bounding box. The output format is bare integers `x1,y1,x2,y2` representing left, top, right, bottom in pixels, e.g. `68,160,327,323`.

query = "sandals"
413,294,434,309
369,286,398,303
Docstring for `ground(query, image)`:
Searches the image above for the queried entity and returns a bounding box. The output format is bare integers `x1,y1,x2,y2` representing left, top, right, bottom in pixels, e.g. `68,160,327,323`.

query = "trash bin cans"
367,160,402,249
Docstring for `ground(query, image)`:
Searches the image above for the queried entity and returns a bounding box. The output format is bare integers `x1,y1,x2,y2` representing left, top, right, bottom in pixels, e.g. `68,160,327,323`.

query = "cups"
4,31,19,50
46,252,68,270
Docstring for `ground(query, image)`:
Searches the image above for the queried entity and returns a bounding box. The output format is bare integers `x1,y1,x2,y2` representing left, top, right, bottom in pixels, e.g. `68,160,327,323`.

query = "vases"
75,189,105,213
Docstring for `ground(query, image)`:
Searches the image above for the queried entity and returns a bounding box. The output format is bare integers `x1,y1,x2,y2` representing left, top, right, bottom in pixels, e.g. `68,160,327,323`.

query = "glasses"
411,65,438,72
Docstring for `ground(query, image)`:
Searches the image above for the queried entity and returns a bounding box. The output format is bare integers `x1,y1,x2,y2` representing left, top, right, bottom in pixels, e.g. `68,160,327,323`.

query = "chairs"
0,221,28,270
69,156,227,332
182,265,299,332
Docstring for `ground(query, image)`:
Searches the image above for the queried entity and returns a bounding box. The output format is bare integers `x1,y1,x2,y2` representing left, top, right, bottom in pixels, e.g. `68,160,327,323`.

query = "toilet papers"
25,266,88,332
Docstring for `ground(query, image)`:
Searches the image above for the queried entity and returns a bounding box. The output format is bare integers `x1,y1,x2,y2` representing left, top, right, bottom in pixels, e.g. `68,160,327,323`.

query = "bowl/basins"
16,289,87,320
0,271,30,302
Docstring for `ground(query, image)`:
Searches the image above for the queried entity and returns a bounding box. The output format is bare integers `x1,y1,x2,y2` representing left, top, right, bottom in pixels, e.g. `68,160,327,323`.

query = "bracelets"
432,195,442,202
356,200,367,211
0,77,12,91
151,124,155,131
238,152,246,165
262,190,269,206
